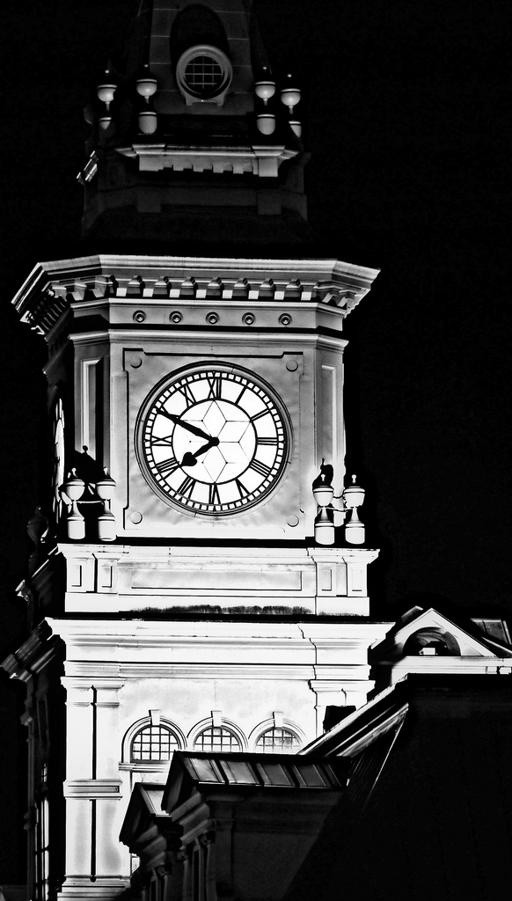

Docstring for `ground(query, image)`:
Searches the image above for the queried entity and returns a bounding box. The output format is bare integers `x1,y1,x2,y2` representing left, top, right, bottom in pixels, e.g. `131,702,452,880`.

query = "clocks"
134,361,294,517
